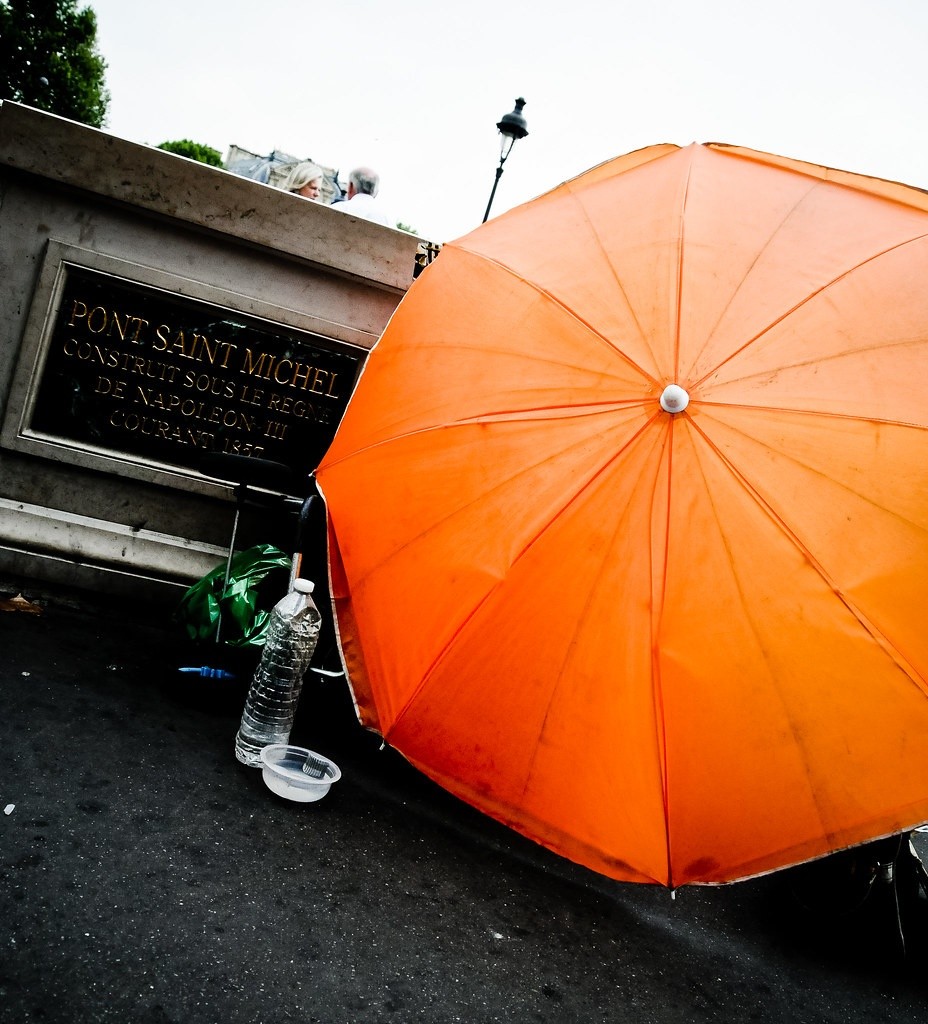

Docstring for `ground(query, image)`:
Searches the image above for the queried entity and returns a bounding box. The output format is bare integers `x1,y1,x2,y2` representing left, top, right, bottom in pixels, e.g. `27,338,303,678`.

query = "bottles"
235,578,322,769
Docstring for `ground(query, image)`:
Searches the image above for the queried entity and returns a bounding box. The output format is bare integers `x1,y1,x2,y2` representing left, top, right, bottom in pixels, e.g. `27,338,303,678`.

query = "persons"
328,167,398,232
281,163,324,199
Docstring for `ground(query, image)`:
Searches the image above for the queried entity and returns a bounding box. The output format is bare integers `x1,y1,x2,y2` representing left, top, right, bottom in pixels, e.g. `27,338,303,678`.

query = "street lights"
482,96,529,223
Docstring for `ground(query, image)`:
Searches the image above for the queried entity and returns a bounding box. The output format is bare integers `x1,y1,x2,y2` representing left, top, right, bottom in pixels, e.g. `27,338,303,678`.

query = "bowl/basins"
260,744,341,802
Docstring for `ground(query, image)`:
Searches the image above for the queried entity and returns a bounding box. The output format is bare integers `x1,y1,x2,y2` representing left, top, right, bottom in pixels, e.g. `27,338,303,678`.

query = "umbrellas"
308,140,927,898
